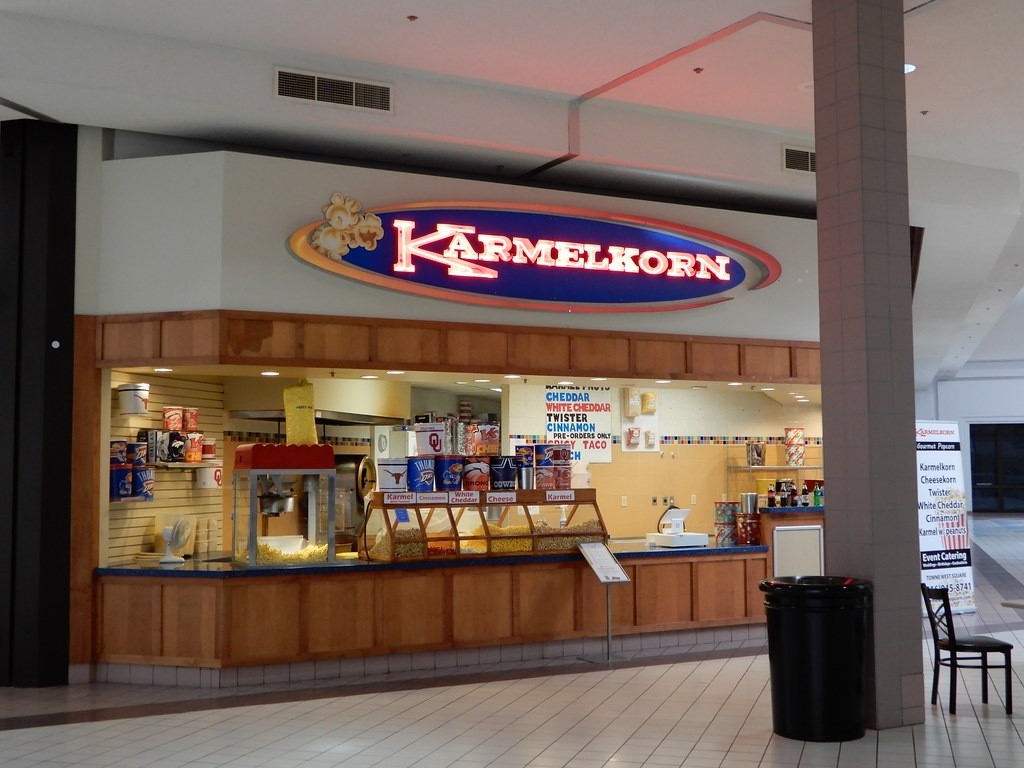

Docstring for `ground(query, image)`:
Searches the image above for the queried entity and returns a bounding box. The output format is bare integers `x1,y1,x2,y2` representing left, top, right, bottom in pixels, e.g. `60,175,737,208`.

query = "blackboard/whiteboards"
772,524,825,578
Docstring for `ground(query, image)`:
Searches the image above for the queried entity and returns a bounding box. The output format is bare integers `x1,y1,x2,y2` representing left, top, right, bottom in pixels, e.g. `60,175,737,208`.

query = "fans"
159,516,192,563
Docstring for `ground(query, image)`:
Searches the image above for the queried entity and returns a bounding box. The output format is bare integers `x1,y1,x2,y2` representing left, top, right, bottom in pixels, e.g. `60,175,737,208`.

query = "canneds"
516,444,572,489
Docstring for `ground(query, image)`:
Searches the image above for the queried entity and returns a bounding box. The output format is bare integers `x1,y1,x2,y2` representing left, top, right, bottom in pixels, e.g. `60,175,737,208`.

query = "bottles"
768,483,776,508
486,413,501,428
814,482,824,506
780,483,787,507
790,482,798,507
802,482,809,506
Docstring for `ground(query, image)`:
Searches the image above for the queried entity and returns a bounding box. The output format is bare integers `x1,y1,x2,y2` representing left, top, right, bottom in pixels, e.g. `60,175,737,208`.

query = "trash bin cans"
756,574,874,743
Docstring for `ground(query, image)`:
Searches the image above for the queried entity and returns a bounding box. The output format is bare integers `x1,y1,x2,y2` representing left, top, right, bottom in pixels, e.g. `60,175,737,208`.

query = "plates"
134,550,183,563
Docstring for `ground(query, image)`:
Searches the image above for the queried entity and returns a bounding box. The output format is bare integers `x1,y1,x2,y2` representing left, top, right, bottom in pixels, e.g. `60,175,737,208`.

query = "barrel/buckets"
116,382,150,416
196,460,224,490
375,455,515,493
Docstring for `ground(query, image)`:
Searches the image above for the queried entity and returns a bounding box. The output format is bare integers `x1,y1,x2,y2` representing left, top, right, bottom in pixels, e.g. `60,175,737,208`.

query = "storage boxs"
196,518,208,530
153,513,197,556
208,539,218,552
147,430,170,462
208,518,218,529
237,534,303,555
194,540,208,554
407,430,445,456
195,529,208,541
208,528,218,540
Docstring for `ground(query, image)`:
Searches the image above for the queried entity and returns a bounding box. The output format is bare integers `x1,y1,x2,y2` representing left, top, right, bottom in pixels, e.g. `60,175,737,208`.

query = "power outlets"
670,496,674,506
662,496,668,506
652,497,658,506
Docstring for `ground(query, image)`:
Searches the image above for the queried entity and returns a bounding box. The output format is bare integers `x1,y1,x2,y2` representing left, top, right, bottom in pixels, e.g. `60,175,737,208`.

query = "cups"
110,438,155,501
196,517,218,553
162,406,217,462
515,445,534,489
535,444,572,489
785,427,806,467
713,493,763,546
744,440,767,466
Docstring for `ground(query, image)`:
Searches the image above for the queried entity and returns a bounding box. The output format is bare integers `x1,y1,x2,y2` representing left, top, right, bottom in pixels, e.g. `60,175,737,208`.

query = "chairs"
921,583,1014,716
